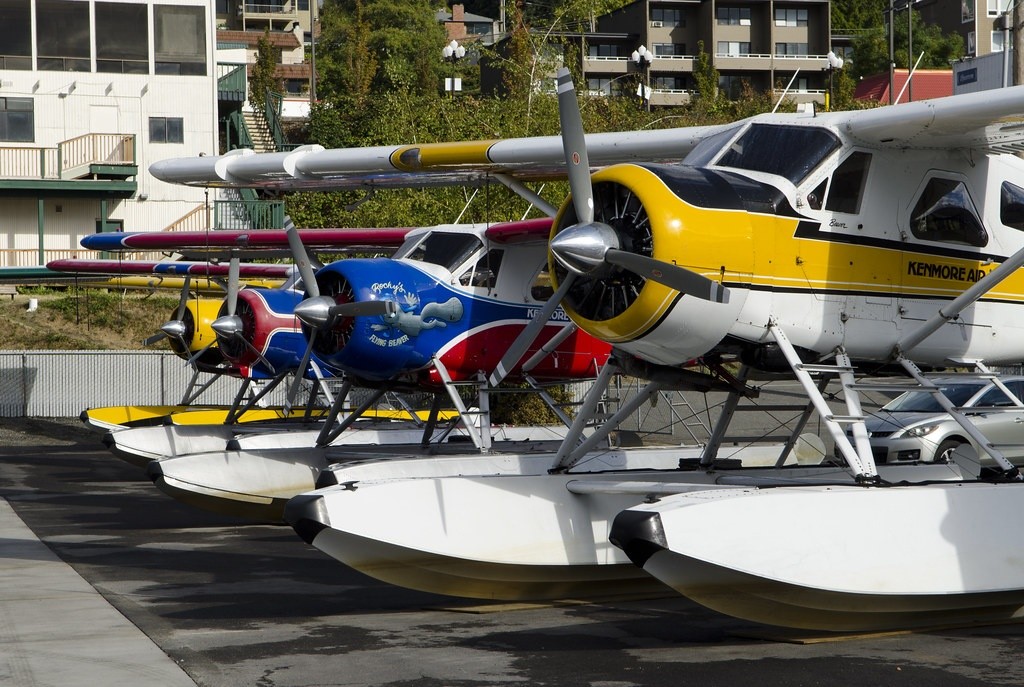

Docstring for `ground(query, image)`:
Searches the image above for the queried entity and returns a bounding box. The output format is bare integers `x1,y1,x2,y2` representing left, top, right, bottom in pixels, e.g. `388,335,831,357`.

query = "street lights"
442,39,466,99
827,51,843,108
632,45,653,111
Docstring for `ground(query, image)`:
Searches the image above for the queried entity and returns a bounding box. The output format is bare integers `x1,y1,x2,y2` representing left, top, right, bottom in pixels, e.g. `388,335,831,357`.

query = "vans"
835,374,1024,468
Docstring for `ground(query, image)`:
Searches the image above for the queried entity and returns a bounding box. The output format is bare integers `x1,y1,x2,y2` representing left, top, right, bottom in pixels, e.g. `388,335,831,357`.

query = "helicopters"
46,67,1024,631
